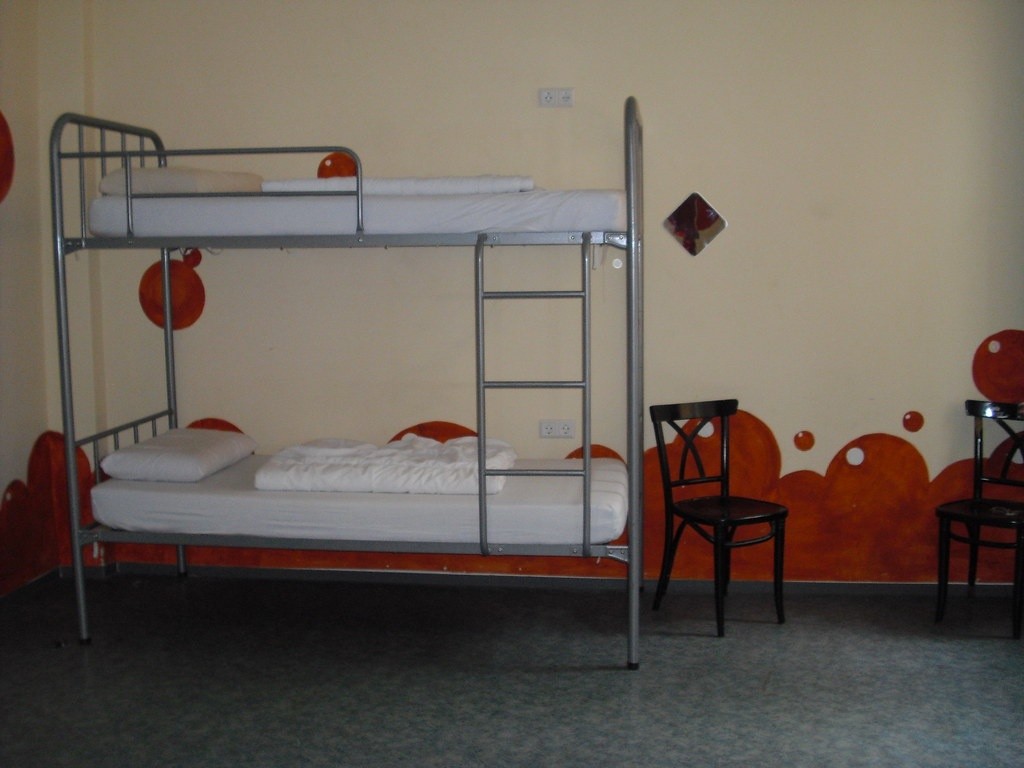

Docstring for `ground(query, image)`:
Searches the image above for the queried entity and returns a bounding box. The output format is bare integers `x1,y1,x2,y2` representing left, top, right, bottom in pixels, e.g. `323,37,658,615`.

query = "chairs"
648,399,790,636
932,399,1024,639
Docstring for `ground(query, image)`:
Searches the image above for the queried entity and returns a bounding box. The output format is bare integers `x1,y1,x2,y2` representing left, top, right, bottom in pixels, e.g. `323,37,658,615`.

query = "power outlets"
539,88,576,108
538,420,578,438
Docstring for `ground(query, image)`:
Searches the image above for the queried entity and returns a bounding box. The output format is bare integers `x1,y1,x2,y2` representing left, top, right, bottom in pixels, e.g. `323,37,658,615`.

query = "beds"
49,96,646,674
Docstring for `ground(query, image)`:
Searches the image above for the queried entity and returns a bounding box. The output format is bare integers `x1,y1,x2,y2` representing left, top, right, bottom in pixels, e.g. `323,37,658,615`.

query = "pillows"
102,429,259,483
97,165,266,196
